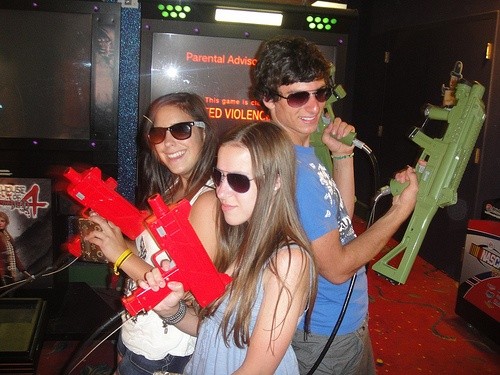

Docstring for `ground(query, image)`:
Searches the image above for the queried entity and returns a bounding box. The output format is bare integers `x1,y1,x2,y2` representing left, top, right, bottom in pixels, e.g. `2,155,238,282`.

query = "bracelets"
160,300,186,334
114,249,134,276
329,153,354,160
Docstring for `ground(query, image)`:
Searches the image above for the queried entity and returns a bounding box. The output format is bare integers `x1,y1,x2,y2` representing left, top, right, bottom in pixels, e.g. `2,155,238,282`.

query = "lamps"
211,5,289,29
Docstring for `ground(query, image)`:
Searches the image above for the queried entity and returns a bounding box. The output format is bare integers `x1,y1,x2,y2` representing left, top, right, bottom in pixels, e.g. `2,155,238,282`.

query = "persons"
83,38,420,375
0,212,26,285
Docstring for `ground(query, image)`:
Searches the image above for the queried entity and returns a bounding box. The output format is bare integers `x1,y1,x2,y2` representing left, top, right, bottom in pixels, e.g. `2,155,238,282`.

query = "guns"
309,63,356,177
371,78,488,285
121,192,234,317
62,166,152,257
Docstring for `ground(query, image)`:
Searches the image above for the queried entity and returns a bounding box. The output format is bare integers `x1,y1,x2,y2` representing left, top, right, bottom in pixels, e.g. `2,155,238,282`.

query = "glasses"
212,166,255,193
148,121,206,144
277,87,332,108
